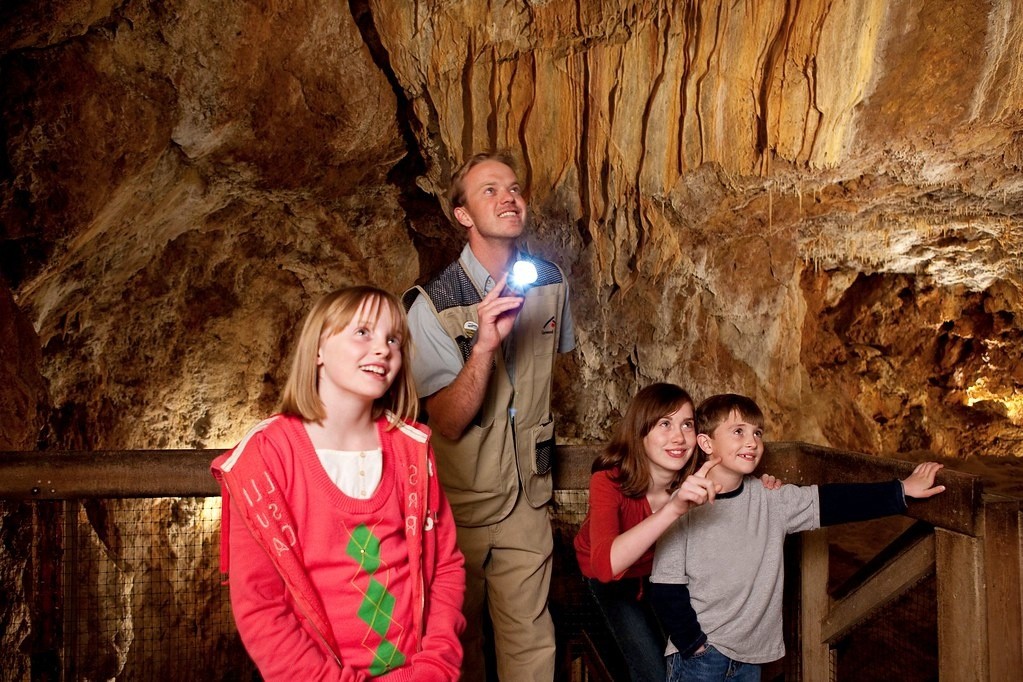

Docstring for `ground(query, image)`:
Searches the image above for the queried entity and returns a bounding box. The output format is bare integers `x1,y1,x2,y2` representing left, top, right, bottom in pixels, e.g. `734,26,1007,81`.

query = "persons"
400,152,574,682
575,382,782,681
649,395,949,682
210,288,468,682
